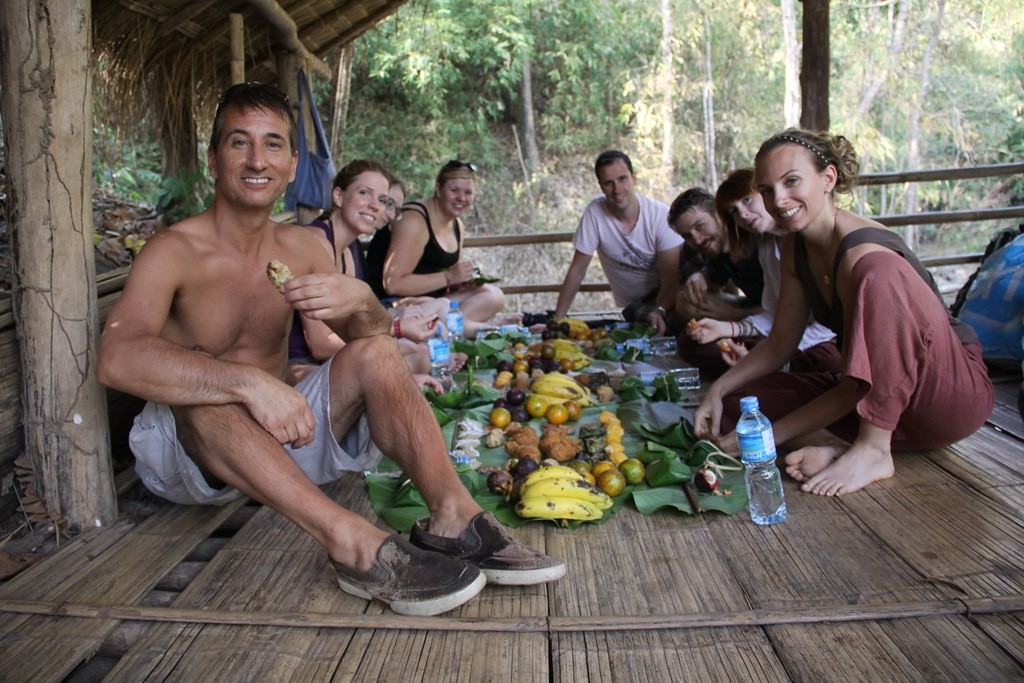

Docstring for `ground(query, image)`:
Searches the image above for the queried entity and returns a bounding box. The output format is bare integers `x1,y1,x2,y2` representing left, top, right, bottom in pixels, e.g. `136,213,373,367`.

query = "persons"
527,148,680,341
692,126,996,497
365,157,521,340
687,166,840,378
91,82,566,615
667,186,767,383
284,157,443,399
342,173,469,374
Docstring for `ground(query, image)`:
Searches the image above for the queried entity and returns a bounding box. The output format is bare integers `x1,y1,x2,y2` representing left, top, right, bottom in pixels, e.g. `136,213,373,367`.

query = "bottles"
736,396,788,525
364,453,469,477
445,301,464,347
631,367,700,390
605,322,635,332
474,324,529,341
616,336,677,358
427,324,453,393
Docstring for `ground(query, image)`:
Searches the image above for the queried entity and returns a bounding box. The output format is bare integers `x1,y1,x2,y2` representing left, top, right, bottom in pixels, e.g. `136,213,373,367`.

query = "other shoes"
328,533,487,616
408,510,566,585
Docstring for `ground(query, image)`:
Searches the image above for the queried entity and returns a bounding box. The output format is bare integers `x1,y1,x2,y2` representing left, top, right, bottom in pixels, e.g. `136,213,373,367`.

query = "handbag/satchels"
957,232,1024,369
950,223,1023,317
285,67,337,211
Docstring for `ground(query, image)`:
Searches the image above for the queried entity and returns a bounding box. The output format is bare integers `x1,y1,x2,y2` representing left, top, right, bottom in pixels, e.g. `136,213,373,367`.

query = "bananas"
527,372,595,407
556,317,589,333
509,465,612,521
543,339,592,370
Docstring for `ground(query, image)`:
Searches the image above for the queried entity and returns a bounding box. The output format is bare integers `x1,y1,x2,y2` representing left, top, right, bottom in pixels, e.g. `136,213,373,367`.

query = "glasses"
211,81,290,142
441,160,477,173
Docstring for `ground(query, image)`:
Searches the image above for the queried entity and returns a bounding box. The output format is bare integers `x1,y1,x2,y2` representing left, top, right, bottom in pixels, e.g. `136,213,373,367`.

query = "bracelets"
653,306,666,315
728,319,754,338
393,319,401,339
443,271,451,292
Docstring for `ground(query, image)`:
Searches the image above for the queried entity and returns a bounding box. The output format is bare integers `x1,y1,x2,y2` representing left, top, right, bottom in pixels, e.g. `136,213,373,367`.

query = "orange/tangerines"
566,457,645,496
512,344,575,374
489,407,511,428
526,395,579,424
574,328,608,341
